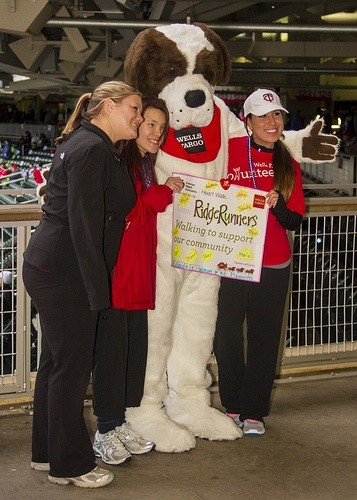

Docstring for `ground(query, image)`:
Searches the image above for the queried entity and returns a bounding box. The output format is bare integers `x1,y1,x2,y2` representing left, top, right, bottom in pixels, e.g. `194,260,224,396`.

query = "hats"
243,88,289,118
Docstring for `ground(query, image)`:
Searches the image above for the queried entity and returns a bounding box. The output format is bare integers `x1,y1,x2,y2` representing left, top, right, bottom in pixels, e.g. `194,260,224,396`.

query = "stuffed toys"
124,20,342,452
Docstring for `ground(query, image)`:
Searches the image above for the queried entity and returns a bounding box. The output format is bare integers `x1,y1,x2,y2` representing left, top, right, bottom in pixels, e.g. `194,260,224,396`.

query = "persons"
21,81,144,489
286,100,356,153
0,129,44,188
1,102,63,125
93,96,187,464
213,89,306,438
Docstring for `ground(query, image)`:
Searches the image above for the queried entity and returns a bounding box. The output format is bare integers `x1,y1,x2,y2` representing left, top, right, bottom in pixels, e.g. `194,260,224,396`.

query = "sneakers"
114,421,156,454
48,465,115,488
243,419,265,434
31,460,51,471
93,430,132,464
225,412,244,428
